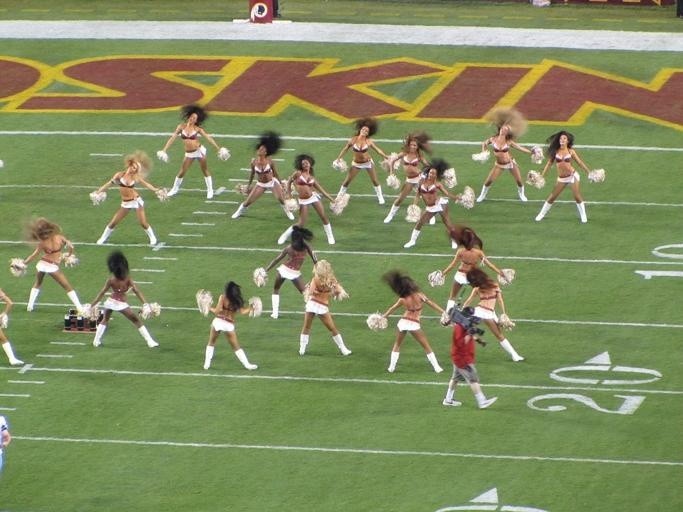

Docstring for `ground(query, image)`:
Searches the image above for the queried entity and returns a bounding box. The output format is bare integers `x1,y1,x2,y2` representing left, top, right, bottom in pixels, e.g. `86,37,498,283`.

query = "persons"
197,282,258,371
478,109,592,223
378,225,524,374
89,151,168,245
0,416,12,477
332,118,391,205
87,252,160,348
0,291,25,366
298,259,353,356
387,134,463,249
443,307,497,407
261,227,319,320
158,105,228,199
13,218,89,312
231,132,338,245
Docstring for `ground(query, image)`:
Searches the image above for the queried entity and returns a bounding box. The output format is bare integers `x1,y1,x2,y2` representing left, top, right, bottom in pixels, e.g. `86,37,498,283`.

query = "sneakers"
478,396,499,409
442,398,463,408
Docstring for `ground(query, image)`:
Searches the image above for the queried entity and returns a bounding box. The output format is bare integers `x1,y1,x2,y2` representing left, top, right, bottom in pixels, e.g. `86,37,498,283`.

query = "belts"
281,261,301,273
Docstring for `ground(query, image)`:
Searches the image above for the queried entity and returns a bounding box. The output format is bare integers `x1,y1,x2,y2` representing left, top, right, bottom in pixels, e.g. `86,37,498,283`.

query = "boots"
499,338,525,363
331,333,352,356
426,351,445,374
298,334,310,355
2,340,25,366
270,295,279,318
476,182,588,224
387,351,400,373
231,181,458,249
203,345,215,369
26,288,159,349
95,175,216,245
233,348,259,371
